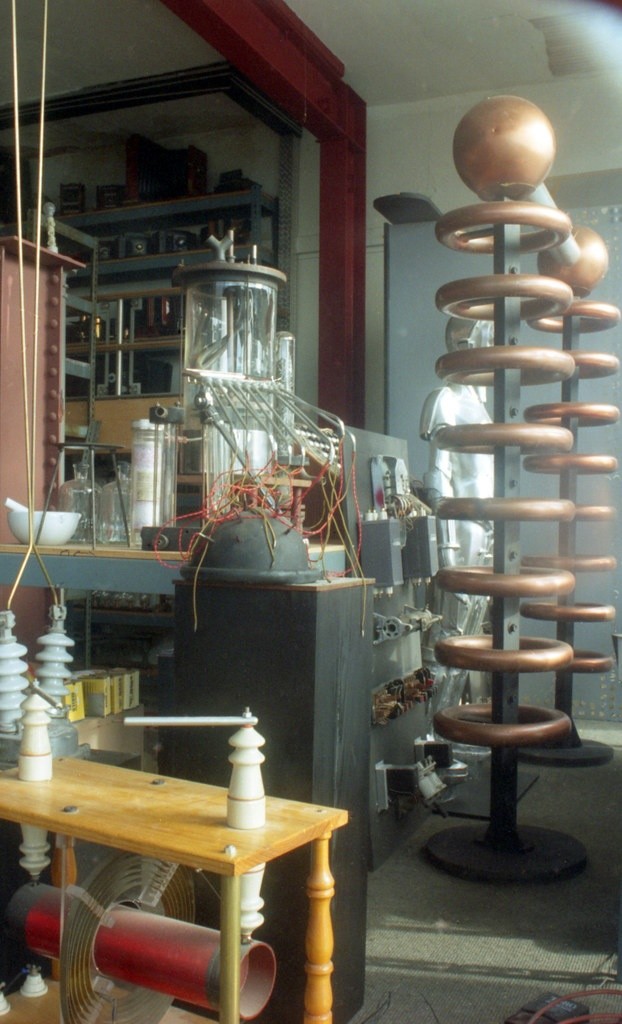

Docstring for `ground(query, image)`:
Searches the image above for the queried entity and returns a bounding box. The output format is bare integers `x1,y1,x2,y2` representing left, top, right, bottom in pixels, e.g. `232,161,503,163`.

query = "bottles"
97,462,135,544
58,462,101,545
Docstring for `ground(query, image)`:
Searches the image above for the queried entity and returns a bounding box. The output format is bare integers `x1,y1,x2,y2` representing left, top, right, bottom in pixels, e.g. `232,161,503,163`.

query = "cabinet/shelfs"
1,190,281,456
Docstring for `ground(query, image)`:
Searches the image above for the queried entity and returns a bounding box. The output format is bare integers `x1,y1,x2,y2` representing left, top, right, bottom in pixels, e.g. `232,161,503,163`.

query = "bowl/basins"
6,510,82,544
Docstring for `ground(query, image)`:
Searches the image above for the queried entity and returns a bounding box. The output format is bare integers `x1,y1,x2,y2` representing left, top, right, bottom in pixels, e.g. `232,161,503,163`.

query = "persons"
415,309,501,778
